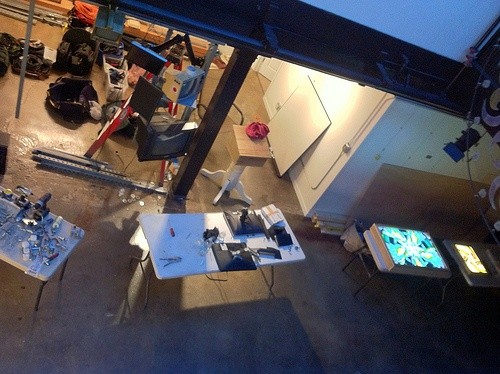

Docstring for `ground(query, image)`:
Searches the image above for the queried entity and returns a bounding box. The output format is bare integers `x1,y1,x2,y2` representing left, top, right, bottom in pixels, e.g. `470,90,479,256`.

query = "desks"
130,208,306,309
342,229,454,309
443,239,500,307
0,185,85,312
199,124,270,206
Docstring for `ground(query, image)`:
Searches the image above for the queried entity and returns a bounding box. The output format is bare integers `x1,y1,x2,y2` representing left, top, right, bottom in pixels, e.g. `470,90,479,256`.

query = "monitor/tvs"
369,223,452,276
129,75,164,124
161,65,205,104
452,242,489,278
125,40,167,77
134,114,197,162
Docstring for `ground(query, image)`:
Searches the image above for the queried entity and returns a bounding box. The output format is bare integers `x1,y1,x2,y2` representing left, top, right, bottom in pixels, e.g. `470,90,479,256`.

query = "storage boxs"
101,54,128,101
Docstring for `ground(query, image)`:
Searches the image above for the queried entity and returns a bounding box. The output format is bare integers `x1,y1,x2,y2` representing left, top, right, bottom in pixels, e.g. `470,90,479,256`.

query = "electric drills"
11,184,53,225
